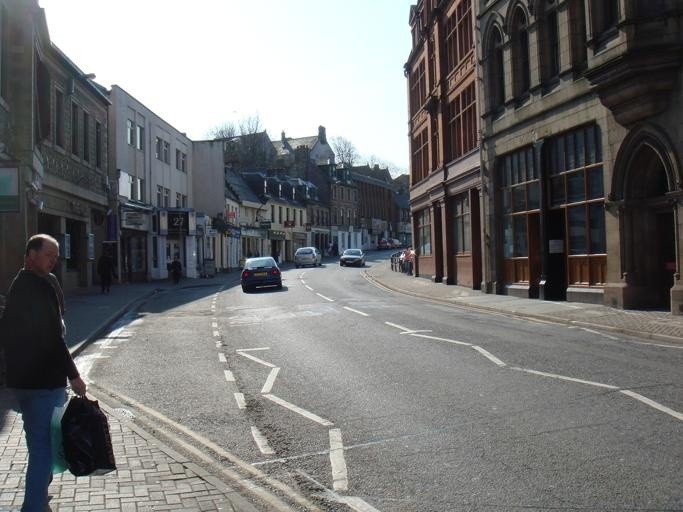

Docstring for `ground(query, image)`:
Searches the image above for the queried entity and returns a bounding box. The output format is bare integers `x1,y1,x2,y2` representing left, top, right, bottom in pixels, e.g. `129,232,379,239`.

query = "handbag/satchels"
51,392,117,477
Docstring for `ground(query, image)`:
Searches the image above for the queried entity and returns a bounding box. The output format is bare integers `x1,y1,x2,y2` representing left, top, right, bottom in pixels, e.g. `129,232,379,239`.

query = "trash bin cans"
204,258,216,278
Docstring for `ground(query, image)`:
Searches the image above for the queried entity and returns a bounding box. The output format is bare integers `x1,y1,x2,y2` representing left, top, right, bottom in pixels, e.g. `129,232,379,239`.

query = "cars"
240,256,281,293
293,246,321,266
378,240,392,249
339,249,367,268
390,238,402,249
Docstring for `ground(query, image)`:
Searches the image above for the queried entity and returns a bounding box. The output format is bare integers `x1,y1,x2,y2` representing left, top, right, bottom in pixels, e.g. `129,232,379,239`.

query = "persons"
96,247,116,294
1,233,86,511
